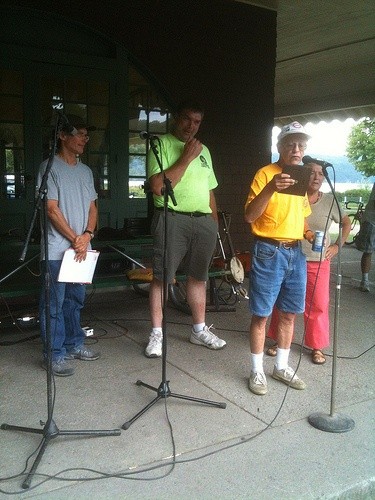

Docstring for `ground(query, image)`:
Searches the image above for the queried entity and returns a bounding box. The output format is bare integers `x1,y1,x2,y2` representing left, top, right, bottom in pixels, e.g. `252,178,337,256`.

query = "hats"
278,121,310,144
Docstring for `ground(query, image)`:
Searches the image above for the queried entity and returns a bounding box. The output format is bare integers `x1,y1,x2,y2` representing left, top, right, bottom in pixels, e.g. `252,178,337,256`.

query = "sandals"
312,348,326,364
266,342,278,356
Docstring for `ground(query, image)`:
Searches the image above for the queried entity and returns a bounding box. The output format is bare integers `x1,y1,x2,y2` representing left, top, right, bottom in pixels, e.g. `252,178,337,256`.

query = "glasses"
280,141,307,150
65,131,89,143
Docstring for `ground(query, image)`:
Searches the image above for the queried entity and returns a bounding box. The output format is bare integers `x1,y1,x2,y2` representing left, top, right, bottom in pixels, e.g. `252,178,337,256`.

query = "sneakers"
41,356,73,376
189,323,226,349
248,369,267,394
361,280,370,292
145,329,163,357
272,363,306,389
64,346,102,361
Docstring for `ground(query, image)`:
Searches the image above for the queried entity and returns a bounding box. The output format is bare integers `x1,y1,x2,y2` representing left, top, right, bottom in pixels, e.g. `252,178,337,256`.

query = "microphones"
139,131,159,140
302,155,332,168
59,110,78,136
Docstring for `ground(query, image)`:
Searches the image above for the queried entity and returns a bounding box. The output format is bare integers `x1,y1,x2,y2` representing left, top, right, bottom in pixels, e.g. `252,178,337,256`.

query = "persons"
34,114,98,376
267,155,351,364
244,121,306,393
357,182,375,292
145,97,227,359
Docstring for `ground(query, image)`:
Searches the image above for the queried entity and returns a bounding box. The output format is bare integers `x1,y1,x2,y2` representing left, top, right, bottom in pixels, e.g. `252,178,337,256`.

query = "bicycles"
170,229,243,312
339,201,366,246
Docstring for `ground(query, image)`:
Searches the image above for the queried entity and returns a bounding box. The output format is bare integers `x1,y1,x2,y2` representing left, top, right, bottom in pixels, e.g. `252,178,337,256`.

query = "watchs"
84,230,95,240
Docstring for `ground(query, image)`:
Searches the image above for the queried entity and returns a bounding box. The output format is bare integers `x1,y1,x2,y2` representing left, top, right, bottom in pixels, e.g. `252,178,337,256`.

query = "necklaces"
313,191,320,204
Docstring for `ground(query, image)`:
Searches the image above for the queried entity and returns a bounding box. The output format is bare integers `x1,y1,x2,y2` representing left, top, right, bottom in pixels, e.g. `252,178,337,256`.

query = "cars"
6,175,25,196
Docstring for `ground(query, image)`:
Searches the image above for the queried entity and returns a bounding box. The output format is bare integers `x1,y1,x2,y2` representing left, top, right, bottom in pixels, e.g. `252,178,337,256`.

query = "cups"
311,231,324,252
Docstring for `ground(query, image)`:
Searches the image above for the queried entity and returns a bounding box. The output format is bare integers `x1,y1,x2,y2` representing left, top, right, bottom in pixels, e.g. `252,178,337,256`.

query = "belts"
154,207,212,217
255,234,300,249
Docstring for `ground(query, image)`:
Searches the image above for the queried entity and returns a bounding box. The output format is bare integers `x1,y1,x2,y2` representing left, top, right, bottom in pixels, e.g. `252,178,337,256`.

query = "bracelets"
304,230,310,239
335,243,339,246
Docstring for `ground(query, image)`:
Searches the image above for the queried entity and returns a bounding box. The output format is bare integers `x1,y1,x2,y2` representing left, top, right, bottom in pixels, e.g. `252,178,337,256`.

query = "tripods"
0,116,122,490
121,138,227,430
